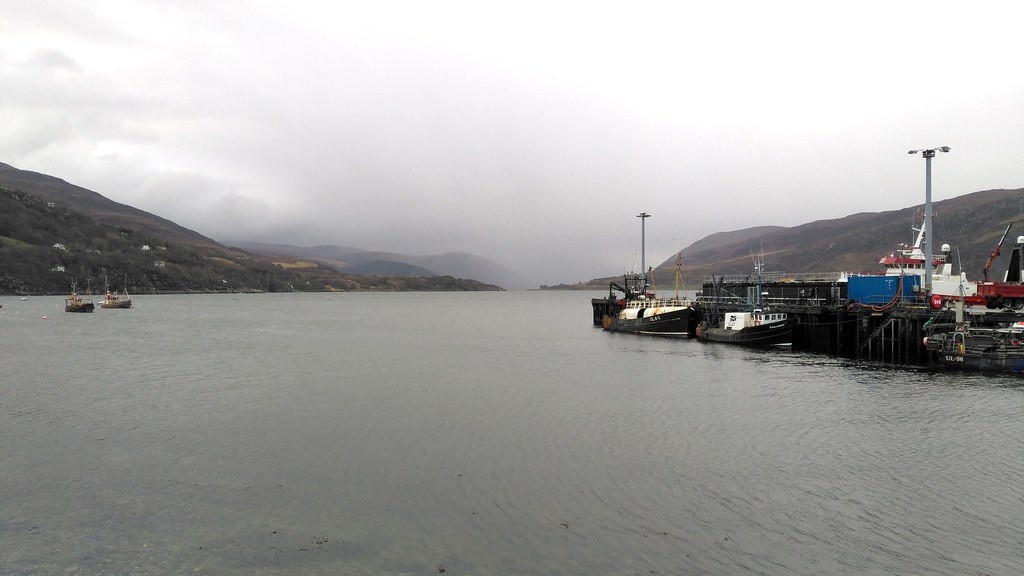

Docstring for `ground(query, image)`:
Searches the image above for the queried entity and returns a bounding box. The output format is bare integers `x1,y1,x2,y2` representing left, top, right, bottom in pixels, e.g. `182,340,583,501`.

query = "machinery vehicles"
977,222,1024,311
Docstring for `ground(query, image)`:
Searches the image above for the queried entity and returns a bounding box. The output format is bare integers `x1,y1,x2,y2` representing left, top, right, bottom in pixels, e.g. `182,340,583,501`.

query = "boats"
97,300,105,305
833,206,998,308
20,297,29,301
922,245,1024,374
602,273,701,342
64,276,95,313
102,274,132,309
696,254,795,347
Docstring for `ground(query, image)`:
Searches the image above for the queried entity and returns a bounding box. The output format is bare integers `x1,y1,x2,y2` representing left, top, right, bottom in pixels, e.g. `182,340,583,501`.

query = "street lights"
636,213,652,295
